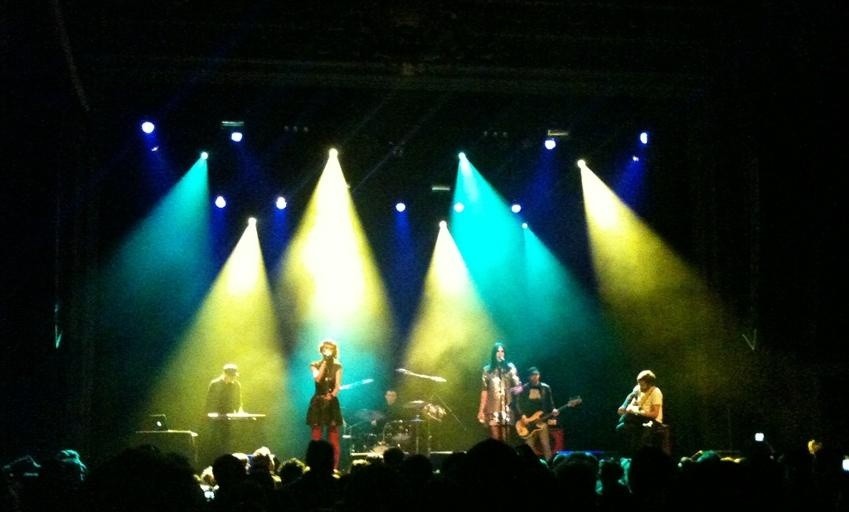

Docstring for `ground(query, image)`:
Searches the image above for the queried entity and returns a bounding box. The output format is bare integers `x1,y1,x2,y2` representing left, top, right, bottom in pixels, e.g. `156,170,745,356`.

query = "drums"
372,431,390,453
385,420,412,441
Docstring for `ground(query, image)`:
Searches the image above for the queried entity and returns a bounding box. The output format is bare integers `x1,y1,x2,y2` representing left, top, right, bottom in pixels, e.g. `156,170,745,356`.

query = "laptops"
151,414,178,431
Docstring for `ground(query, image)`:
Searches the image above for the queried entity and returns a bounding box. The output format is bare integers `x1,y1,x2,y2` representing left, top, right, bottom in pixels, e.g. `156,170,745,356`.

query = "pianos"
205,412,268,422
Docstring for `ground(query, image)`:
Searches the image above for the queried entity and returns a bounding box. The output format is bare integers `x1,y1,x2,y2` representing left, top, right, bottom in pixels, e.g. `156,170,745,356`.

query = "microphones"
500,357,505,361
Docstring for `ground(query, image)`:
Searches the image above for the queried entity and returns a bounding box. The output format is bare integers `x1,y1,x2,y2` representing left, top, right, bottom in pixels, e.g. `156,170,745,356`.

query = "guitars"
515,395,583,439
624,394,646,416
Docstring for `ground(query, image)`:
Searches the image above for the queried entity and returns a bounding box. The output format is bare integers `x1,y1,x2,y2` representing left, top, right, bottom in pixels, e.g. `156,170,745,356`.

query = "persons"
477,341,523,444
304,339,344,471
366,389,400,436
194,363,246,466
0,434,849,512
614,368,666,459
514,364,561,461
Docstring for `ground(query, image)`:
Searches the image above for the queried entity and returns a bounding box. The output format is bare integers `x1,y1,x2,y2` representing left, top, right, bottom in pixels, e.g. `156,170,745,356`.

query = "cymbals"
353,408,384,422
403,400,427,410
